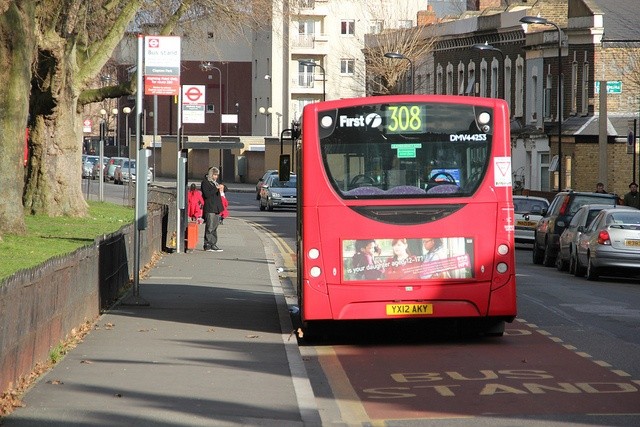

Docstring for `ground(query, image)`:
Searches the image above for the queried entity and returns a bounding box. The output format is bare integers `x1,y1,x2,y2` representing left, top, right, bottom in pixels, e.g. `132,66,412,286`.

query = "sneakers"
204,244,215,252
212,244,223,252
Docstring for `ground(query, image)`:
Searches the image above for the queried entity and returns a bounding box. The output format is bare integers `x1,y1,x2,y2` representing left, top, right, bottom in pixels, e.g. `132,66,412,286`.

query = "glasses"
598,187,603,189
423,240,432,243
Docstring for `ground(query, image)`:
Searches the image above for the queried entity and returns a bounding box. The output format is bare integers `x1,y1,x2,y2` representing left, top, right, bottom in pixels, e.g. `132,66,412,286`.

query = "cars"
104,156,129,180
256,169,294,199
556,203,633,268
82,155,108,177
260,174,297,209
114,158,154,186
92,158,110,180
575,208,640,279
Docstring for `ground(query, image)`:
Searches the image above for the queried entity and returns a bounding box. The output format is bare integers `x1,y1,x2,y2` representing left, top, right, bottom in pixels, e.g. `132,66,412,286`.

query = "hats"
629,182,638,188
206,167,219,182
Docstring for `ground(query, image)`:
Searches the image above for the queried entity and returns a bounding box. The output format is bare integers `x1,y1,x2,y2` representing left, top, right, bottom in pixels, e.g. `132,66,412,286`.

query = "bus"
279,93,517,334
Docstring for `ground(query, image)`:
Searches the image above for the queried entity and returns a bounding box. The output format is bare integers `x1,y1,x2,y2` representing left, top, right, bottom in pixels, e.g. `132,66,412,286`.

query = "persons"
384,239,421,280
595,183,609,194
218,189,229,226
422,239,454,282
188,181,204,225
624,182,640,210
350,239,382,281
201,167,224,252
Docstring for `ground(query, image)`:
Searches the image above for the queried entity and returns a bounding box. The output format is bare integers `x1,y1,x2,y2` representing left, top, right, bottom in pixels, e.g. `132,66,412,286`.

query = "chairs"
426,184,461,193
347,186,383,195
385,186,426,194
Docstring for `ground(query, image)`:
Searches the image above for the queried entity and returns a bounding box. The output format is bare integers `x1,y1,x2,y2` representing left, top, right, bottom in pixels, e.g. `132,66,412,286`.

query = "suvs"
512,194,551,243
533,188,624,264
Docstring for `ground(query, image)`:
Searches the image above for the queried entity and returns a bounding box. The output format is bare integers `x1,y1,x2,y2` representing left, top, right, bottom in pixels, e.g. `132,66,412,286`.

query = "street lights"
472,44,505,99
384,52,414,94
199,64,222,184
519,16,562,191
259,106,273,135
100,109,106,122
101,75,119,108
300,61,325,101
112,108,119,146
123,106,131,146
